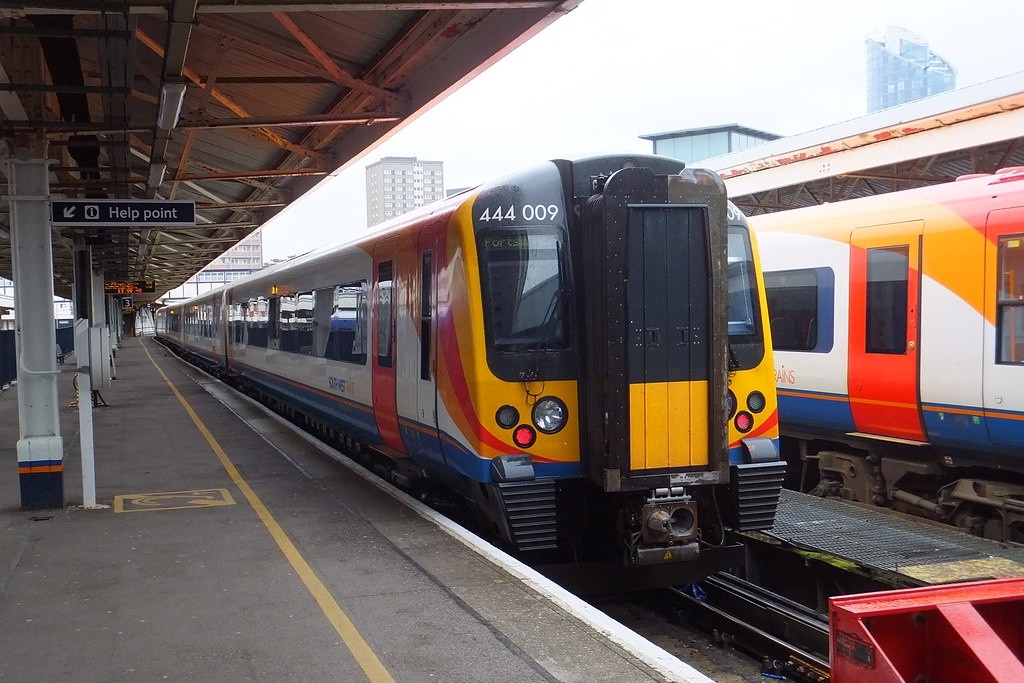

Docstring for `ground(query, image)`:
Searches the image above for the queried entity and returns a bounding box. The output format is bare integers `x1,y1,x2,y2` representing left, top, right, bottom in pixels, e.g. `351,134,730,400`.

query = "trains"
154,153,789,592
746,166,1024,542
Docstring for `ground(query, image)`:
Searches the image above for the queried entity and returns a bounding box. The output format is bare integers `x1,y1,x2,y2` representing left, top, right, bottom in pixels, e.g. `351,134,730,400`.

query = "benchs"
56,344,66,365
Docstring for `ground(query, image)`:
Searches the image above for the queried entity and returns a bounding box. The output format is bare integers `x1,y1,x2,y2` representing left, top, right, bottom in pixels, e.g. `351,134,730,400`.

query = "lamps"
156,82,187,130
